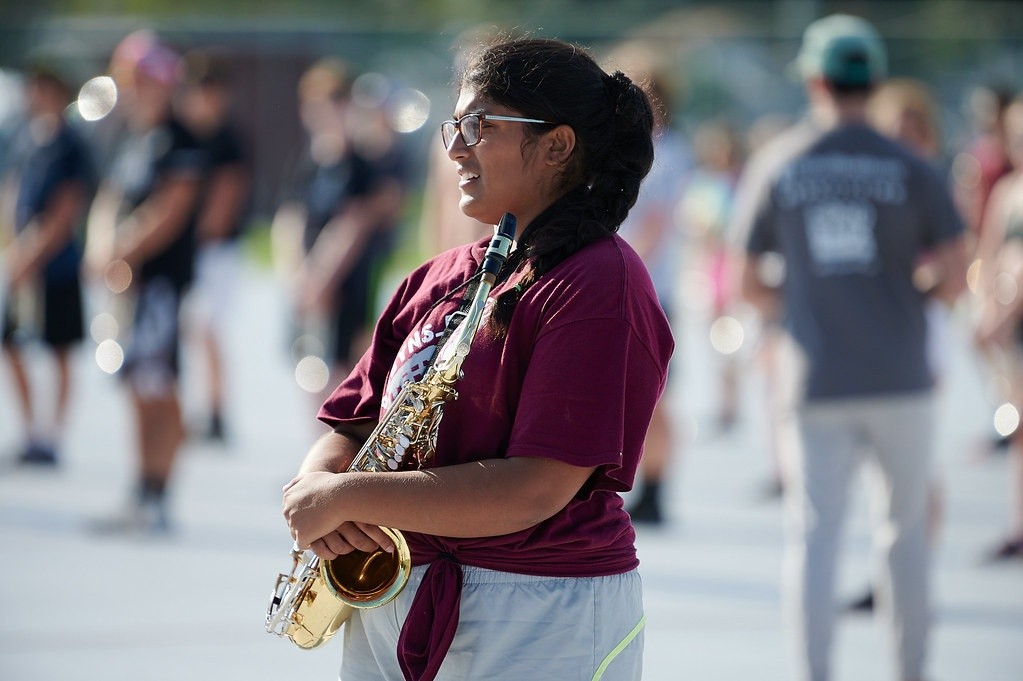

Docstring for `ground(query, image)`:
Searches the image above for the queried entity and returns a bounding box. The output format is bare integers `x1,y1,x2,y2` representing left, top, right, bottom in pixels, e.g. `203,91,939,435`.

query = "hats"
798,14,890,85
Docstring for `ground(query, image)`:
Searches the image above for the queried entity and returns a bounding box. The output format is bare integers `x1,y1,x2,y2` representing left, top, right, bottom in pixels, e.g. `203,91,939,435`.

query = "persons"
0,30,496,530
620,16,1022,680
283,39,675,681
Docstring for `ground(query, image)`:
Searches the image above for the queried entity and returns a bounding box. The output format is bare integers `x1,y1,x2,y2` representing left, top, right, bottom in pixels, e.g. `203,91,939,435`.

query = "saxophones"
267,210,522,650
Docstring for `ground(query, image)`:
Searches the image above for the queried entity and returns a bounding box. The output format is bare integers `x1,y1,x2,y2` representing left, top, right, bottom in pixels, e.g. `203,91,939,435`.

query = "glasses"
440,110,554,150
